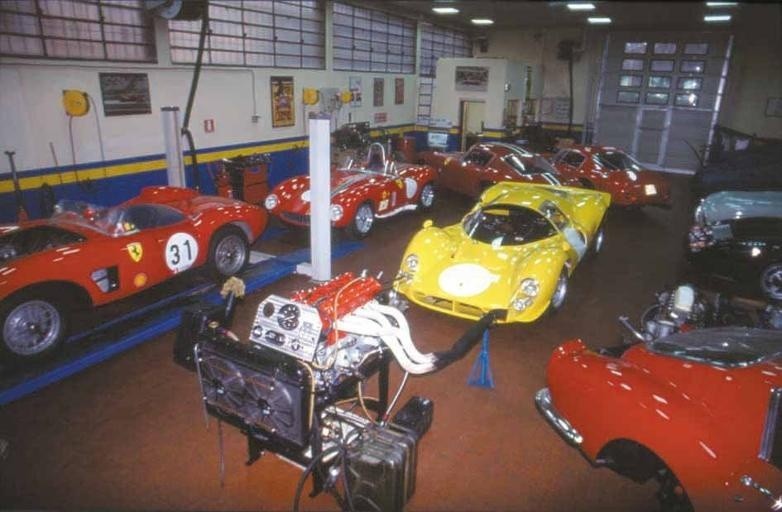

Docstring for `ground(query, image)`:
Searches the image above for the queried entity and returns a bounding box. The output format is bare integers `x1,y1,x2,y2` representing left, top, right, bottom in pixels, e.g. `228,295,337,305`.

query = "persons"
706,124,723,160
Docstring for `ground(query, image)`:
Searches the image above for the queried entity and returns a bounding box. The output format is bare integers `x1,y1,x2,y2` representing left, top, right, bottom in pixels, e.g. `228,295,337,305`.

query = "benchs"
113,202,183,234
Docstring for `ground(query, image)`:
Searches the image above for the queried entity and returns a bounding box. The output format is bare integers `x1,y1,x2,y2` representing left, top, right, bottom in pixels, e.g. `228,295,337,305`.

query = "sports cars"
264,143,438,238
682,188,782,306
421,138,578,217
388,180,613,328
533,142,670,212
0,180,271,373
533,324,782,509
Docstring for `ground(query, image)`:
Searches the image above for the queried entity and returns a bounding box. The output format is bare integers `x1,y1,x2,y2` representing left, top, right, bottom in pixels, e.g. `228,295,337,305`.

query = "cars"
690,144,782,193
